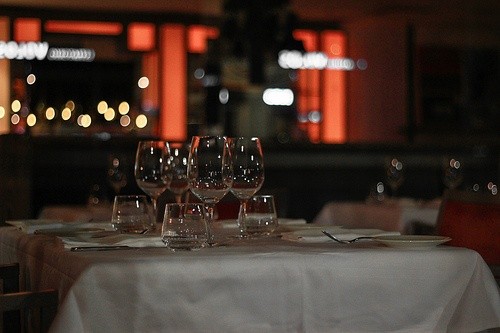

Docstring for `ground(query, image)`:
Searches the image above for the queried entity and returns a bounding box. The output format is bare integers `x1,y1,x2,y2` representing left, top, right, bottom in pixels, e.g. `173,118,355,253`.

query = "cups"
111,195,157,236
441,155,500,201
244,194,279,240
364,177,385,207
161,202,209,253
87,184,110,214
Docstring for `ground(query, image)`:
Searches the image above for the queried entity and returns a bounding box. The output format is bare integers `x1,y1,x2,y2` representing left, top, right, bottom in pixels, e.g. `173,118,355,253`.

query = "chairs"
436,191,500,264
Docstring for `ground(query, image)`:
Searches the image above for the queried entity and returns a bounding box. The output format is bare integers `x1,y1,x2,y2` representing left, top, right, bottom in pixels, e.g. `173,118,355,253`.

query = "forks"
322,230,373,245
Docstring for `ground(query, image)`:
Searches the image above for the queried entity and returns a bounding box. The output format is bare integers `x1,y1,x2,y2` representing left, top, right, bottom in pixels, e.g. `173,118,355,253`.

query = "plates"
371,235,454,249
5,220,64,226
279,223,344,231
36,227,106,236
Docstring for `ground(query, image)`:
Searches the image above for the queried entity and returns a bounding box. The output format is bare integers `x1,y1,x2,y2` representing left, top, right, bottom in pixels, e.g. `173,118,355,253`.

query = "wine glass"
107,152,129,197
384,153,407,208
221,135,266,239
166,142,197,203
186,136,233,249
134,140,174,232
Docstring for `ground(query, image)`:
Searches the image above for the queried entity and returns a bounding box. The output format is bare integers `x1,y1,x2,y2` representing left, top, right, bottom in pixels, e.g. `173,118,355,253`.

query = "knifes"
71,245,167,251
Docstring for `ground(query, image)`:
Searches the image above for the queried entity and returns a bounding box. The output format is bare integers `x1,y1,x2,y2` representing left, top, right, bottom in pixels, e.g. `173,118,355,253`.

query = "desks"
0,223,500,333
40,202,156,222
312,200,500,293
